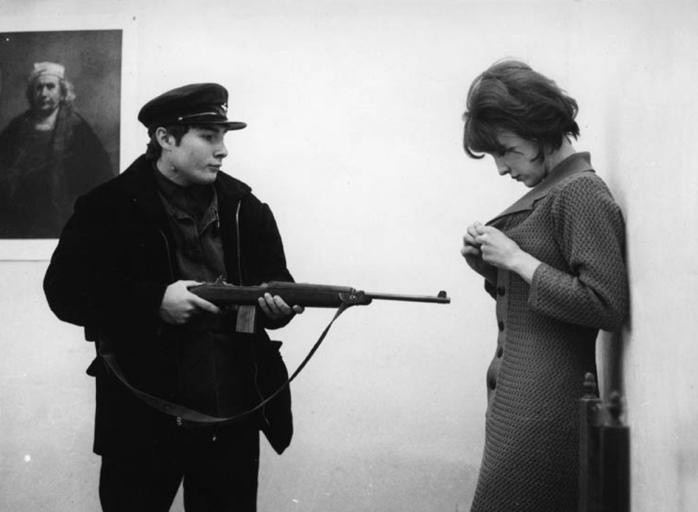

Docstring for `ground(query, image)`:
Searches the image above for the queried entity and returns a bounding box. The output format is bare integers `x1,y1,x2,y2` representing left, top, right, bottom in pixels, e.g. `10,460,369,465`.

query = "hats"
27,63,66,81
138,84,246,138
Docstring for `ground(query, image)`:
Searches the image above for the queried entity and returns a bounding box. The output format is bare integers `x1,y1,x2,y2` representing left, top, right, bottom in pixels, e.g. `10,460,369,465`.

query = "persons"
43,81,304,512
460,60,631,512
0,60,112,238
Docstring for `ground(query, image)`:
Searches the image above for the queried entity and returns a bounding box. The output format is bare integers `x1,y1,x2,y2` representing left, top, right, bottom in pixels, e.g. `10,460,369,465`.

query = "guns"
85,274,450,377
